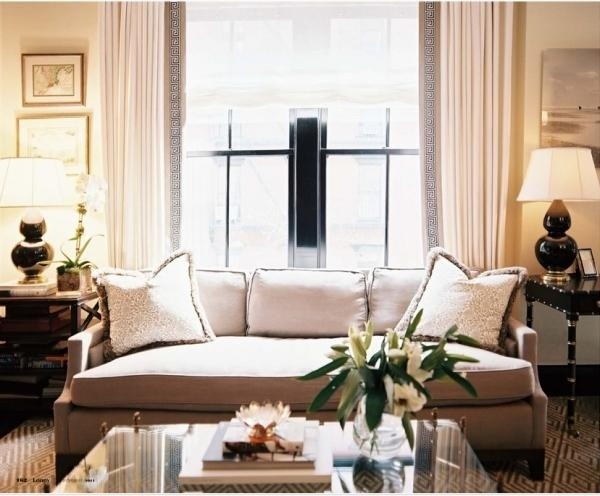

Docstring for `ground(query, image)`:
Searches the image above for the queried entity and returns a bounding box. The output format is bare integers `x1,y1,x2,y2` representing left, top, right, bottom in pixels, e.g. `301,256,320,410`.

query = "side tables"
525,273,600,436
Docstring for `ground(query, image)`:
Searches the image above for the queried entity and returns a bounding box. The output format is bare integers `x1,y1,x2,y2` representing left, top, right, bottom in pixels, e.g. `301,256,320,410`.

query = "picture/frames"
22,53,84,105
576,248,599,279
576,248,597,278
15,113,91,177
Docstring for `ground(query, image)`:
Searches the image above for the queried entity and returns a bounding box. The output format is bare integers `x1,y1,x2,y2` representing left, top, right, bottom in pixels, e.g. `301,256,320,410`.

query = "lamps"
516,146,599,286
0,157,78,285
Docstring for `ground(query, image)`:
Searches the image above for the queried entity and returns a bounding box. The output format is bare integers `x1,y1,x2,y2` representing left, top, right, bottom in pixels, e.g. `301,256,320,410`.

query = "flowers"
294,308,479,452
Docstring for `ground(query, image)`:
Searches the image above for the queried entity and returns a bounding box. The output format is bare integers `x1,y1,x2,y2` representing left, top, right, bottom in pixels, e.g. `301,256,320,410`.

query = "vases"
353,394,407,452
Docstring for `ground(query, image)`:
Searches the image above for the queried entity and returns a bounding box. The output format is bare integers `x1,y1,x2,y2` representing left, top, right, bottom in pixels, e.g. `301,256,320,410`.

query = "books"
181,417,333,487
1,282,72,399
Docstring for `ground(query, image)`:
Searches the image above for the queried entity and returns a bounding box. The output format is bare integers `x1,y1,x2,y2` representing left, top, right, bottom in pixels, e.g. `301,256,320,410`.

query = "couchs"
53,247,548,483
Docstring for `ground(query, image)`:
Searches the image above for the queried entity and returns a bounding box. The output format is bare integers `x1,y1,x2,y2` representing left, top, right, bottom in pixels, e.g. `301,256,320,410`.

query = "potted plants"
57,203,105,291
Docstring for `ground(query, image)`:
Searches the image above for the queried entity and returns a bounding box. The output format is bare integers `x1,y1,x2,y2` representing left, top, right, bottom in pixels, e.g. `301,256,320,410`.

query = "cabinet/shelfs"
1,285,101,423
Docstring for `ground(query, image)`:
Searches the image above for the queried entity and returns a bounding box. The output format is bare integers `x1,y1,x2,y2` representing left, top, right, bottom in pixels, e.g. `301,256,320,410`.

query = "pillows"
92,251,214,361
140,269,249,337
390,249,528,354
369,267,485,335
245,269,368,337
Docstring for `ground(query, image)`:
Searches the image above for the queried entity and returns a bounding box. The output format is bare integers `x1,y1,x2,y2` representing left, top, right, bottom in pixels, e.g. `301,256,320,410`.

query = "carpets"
0,392,599,493
